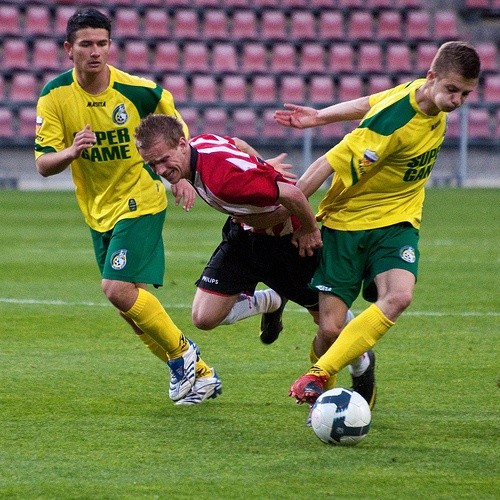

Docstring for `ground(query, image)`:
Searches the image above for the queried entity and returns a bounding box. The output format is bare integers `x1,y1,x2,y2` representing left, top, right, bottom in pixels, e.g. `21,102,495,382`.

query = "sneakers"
288,371,330,405
174,368,224,406
306,403,314,426
259,291,288,345
349,350,377,410
166,337,200,401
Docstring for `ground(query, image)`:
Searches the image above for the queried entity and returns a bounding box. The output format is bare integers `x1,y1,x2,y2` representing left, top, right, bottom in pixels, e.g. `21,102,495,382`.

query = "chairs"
0,0,500,152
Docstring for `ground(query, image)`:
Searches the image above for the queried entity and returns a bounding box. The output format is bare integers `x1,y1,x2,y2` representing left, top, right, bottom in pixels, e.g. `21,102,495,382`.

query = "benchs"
429,170,459,186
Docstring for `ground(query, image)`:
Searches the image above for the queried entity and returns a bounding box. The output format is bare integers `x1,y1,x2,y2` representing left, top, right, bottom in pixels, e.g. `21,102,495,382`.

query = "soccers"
311,388,372,445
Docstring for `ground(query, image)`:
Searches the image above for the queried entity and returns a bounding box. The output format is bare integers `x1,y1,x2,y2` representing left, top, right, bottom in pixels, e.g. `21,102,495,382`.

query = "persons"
35,9,222,405
133,116,374,412
244,41,480,405
